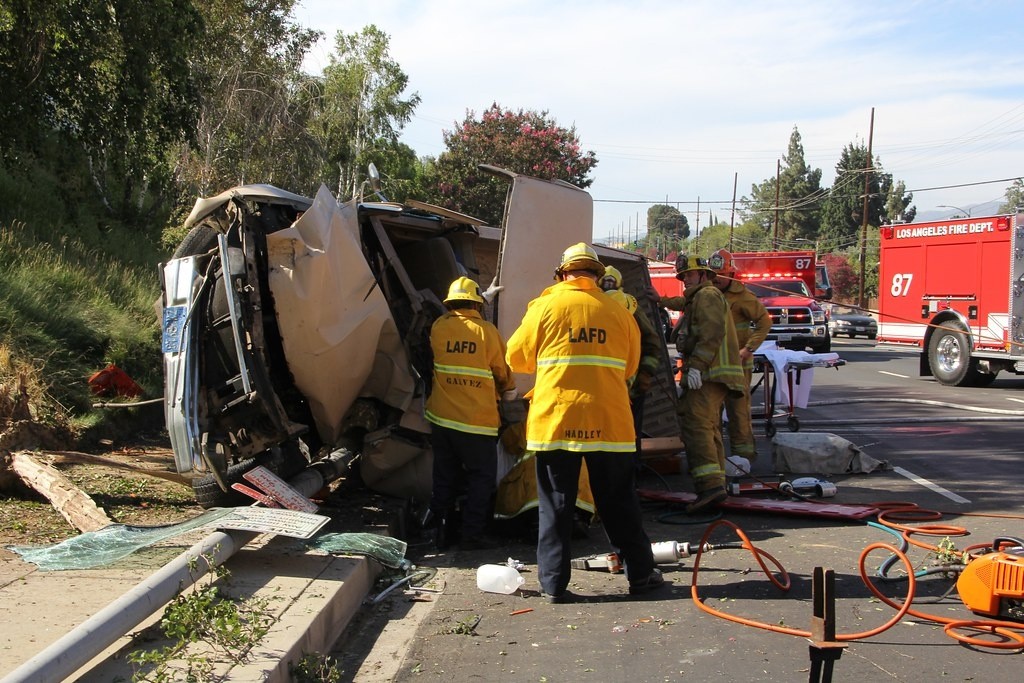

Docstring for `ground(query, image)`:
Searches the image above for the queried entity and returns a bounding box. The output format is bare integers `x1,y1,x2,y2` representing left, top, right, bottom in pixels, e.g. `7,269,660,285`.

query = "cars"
821,303,878,340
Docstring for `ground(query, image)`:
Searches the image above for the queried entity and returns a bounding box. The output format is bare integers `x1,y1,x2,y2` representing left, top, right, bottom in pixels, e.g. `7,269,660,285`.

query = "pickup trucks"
156,163,675,512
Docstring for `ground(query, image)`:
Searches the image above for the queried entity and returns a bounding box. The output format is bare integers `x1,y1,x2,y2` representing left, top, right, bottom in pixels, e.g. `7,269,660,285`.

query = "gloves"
502,389,517,401
482,276,505,304
688,368,703,391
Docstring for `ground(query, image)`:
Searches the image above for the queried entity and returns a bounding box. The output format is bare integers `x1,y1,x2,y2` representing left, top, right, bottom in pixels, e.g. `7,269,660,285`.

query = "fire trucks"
872,208,1024,388
647,250,832,372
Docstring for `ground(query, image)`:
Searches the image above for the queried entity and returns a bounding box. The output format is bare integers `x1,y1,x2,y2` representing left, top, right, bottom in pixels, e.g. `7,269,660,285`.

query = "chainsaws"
555,519,791,591
725,472,837,501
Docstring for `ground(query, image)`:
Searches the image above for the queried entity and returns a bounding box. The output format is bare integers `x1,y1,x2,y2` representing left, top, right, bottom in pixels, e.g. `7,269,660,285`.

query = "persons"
675,254,747,516
504,243,664,602
599,265,662,467
644,249,772,464
425,276,517,549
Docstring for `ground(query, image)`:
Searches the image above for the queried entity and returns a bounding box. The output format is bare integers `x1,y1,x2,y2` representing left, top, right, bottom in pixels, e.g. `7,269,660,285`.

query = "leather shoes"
538,585,561,603
629,568,664,595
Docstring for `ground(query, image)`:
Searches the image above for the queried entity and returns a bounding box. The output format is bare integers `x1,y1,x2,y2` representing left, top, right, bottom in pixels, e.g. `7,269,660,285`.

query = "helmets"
443,276,484,304
675,255,716,281
598,266,622,288
554,243,606,280
708,249,738,274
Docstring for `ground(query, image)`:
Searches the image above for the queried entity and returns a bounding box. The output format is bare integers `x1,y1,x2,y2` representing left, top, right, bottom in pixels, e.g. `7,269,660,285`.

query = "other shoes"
686,485,727,515
460,534,501,550
